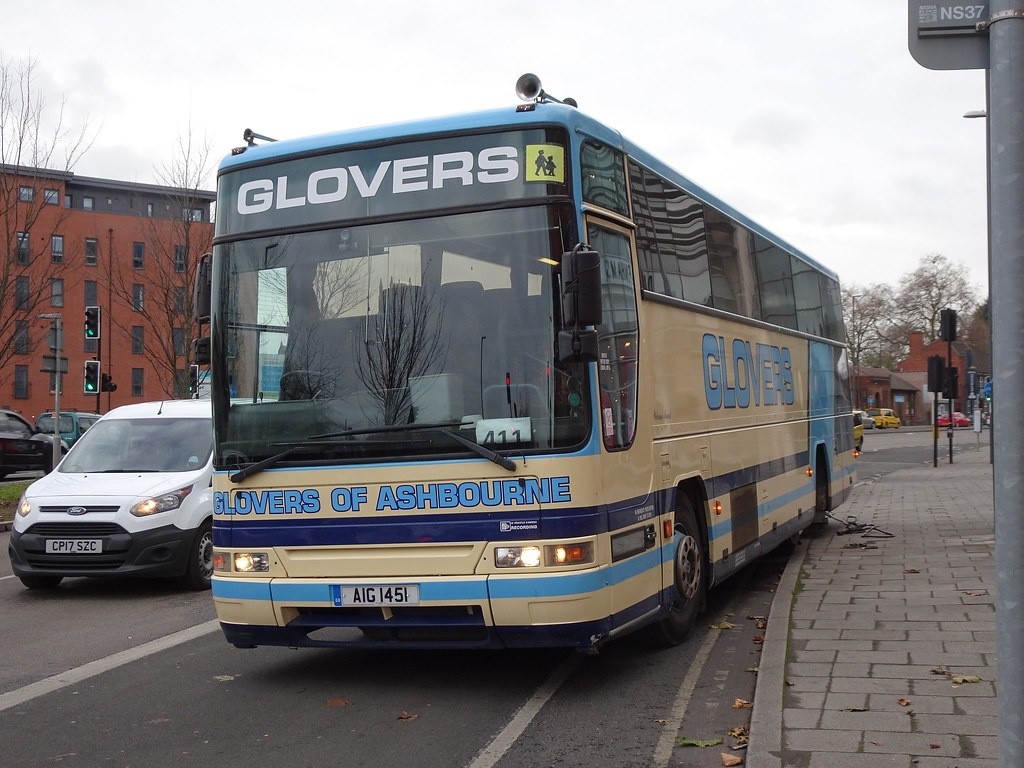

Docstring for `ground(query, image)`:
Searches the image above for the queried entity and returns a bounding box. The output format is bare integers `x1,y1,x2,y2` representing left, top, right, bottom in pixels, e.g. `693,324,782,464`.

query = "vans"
853,413,863,450
35,412,102,448
9,399,282,591
866,408,901,429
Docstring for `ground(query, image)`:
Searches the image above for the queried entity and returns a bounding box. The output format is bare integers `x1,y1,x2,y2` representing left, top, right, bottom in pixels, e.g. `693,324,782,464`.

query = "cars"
853,411,877,429
937,412,971,427
0,410,69,480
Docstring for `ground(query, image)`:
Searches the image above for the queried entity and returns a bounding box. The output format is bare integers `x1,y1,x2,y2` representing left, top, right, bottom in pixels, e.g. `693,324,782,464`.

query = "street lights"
849,294,860,411
481,336,487,418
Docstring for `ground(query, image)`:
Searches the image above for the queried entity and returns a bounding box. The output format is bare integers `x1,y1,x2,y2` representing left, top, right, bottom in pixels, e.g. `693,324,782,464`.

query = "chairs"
485,287,521,332
522,295,548,329
439,281,492,347
380,286,427,313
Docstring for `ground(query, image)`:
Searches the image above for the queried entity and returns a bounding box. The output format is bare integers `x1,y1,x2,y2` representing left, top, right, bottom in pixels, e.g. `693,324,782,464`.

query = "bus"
191,73,860,657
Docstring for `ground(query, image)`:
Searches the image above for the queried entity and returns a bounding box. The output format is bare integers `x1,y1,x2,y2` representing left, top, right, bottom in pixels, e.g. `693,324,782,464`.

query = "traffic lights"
561,370,581,417
189,365,197,392
85,361,99,394
506,372,510,386
986,395,991,401
102,374,117,392
84,306,100,339
